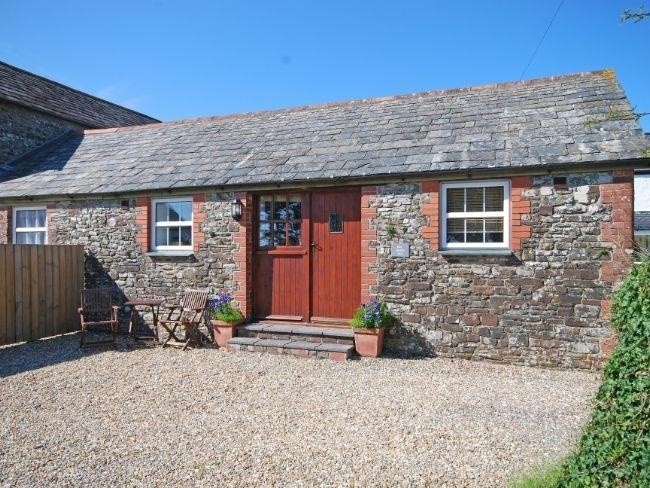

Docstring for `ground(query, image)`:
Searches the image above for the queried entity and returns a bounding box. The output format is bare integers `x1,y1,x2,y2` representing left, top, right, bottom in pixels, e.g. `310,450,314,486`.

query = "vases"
209,319,243,346
353,327,384,356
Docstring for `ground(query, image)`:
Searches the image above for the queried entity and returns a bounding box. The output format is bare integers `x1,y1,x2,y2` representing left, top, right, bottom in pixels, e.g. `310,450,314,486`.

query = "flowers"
350,296,392,329
209,293,244,322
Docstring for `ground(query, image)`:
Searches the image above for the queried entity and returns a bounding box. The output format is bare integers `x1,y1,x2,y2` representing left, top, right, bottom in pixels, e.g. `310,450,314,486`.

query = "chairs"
79,288,210,351
